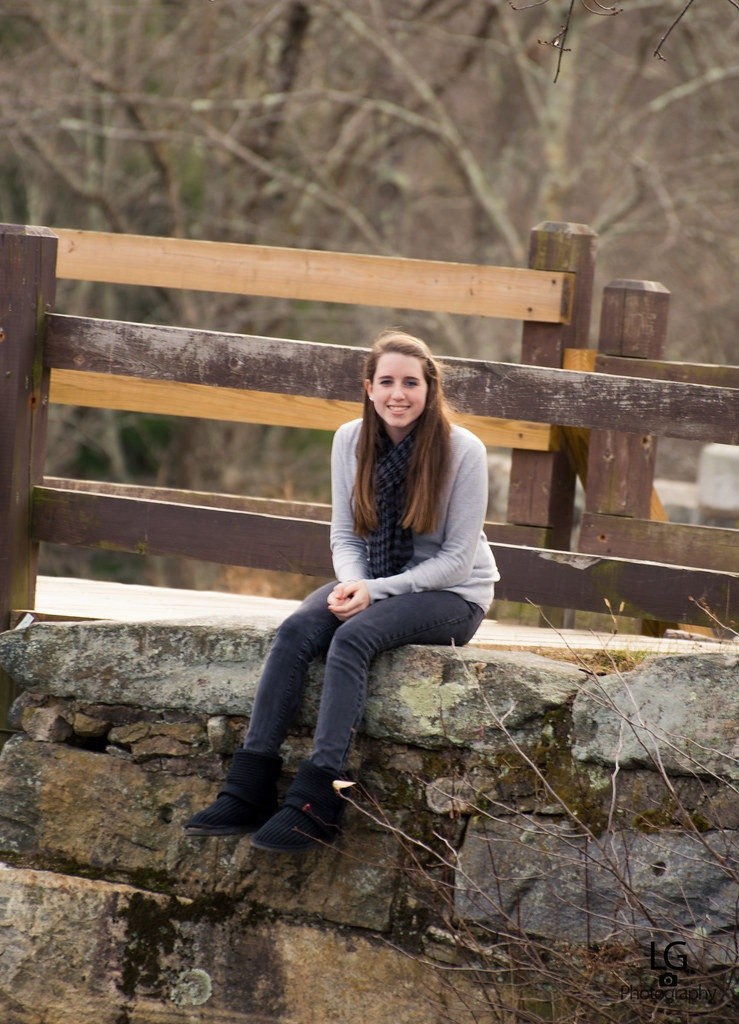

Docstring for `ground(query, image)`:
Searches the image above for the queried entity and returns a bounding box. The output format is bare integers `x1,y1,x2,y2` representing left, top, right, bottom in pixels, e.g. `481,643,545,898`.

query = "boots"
248,759,350,854
184,749,283,836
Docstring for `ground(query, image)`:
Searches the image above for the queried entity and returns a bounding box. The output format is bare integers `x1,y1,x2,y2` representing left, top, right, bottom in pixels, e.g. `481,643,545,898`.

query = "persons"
183,329,501,854
